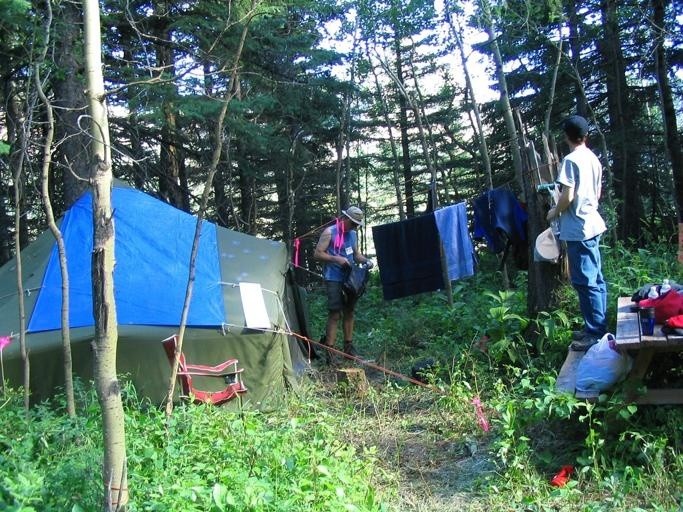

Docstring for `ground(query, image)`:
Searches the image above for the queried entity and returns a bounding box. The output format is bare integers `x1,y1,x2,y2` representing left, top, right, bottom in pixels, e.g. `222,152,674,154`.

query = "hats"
341,206,365,227
561,115,589,138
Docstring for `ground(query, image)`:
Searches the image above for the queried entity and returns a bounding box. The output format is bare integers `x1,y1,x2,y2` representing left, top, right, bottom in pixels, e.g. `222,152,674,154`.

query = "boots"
343,340,365,362
319,336,343,366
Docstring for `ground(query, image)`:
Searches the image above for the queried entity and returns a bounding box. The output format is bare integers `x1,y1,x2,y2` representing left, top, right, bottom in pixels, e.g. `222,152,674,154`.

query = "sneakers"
571,329,601,352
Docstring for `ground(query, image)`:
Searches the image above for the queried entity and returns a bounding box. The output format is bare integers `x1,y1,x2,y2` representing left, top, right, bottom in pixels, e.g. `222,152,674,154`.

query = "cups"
640,308,655,336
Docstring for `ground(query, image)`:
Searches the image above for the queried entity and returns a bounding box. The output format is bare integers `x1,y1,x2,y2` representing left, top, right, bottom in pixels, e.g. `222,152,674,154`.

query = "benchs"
555,340,602,411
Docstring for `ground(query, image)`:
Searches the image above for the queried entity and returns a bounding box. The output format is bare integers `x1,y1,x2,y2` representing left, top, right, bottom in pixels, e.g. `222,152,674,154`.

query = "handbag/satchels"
533,225,563,265
342,262,370,300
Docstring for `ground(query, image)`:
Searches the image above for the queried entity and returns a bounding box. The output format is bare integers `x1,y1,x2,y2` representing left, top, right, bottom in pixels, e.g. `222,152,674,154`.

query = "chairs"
161,334,247,422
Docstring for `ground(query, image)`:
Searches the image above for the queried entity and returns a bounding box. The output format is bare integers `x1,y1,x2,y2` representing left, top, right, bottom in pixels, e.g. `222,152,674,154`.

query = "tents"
0,179,323,420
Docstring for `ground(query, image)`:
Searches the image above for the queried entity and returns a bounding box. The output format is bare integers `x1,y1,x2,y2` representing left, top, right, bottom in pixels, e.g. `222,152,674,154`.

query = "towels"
434,201,479,284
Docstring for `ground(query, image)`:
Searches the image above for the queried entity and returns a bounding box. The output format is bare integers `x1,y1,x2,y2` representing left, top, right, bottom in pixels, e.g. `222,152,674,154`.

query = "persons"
545,114,611,353
312,205,373,368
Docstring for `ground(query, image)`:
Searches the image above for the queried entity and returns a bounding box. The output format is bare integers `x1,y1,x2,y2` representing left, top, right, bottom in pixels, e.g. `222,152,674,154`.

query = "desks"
551,286,683,443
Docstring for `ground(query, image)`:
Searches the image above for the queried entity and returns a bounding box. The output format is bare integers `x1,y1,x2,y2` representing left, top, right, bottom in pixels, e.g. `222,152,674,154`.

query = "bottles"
647,278,671,299
